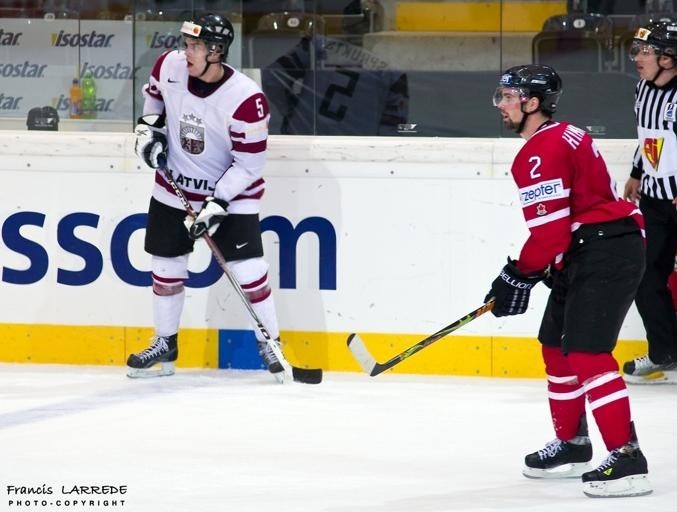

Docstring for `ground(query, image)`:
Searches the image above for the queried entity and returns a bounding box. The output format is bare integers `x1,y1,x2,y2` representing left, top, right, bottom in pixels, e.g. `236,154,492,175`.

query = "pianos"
157,153,321,384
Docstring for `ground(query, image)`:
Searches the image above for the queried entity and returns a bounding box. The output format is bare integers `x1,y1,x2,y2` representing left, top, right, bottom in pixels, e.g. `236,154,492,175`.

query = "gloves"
482,254,549,319
182,195,229,240
131,123,169,169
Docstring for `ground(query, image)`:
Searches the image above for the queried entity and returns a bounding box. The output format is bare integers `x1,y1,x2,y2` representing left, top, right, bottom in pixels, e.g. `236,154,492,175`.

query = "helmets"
178,12,235,63
629,16,677,67
491,64,564,113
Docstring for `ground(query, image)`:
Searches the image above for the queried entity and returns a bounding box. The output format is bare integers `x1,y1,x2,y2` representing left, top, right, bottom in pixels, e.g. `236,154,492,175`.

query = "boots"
524,412,593,469
580,419,648,483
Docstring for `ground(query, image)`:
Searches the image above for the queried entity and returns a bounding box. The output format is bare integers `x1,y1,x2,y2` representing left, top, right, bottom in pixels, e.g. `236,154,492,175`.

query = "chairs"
532,0,677,75
0,0,374,71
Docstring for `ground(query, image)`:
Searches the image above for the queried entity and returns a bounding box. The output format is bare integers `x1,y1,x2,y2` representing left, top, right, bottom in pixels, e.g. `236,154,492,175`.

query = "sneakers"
125,331,180,369
622,353,677,376
256,336,286,374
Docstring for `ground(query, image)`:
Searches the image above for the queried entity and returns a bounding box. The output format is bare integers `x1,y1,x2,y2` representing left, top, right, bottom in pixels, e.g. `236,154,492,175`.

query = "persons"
261,0,413,136
622,18,677,377
485,65,647,483
125,12,288,374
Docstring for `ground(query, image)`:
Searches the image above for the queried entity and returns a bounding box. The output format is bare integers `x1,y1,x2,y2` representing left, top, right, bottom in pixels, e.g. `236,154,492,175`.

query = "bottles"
81,71,96,120
68,78,80,120
